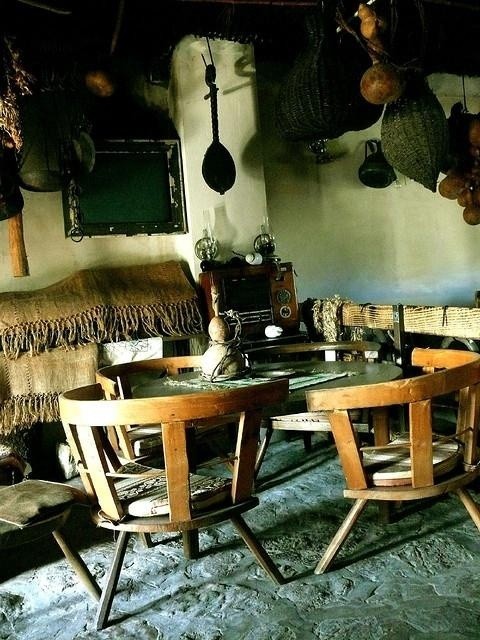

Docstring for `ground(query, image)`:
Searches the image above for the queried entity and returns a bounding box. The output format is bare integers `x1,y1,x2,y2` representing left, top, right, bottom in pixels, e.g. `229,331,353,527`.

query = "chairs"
305,346,480,574
244,341,381,494
0,458,102,599
57,378,290,632
96,355,241,546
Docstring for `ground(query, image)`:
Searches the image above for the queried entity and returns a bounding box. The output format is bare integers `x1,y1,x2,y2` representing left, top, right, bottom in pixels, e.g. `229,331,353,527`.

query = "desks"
171,361,403,493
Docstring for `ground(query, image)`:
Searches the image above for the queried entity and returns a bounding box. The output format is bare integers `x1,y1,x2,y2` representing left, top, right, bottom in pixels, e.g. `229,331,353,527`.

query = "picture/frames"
63,139,187,239
